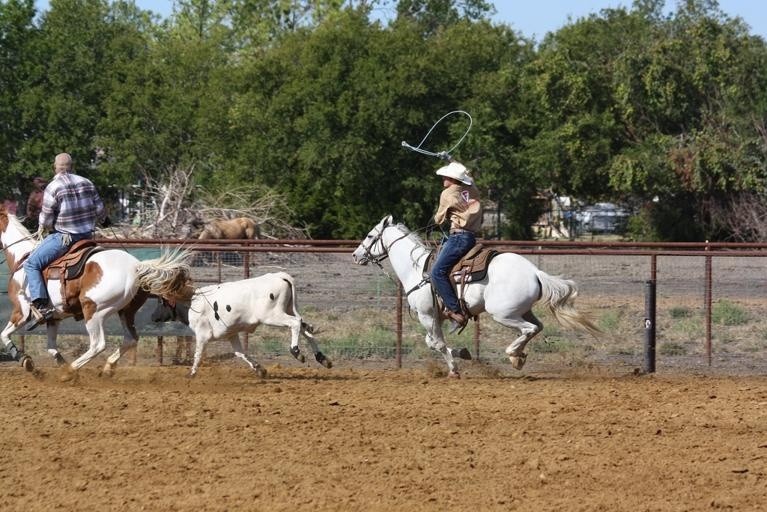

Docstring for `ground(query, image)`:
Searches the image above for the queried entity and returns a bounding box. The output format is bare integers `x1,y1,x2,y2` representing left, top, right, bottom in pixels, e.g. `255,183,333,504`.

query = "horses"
0,199,194,383
351,215,605,380
187,217,258,268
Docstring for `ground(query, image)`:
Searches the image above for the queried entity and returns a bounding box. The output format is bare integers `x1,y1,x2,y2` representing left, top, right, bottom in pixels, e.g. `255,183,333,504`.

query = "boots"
448,311,469,335
26,299,53,331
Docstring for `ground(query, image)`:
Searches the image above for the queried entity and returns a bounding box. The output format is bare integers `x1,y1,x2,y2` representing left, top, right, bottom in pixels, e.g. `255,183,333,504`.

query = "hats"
436,162,473,186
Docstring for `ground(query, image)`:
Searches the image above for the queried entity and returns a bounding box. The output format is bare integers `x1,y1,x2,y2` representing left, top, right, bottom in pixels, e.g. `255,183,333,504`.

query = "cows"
150,272,333,379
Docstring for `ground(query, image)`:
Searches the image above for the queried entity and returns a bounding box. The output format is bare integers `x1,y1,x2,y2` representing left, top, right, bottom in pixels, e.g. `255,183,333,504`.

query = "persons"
24,153,108,330
432,162,482,335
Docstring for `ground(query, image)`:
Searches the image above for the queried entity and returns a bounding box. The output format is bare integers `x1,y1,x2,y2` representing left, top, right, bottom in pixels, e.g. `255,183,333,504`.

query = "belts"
450,228,471,234
